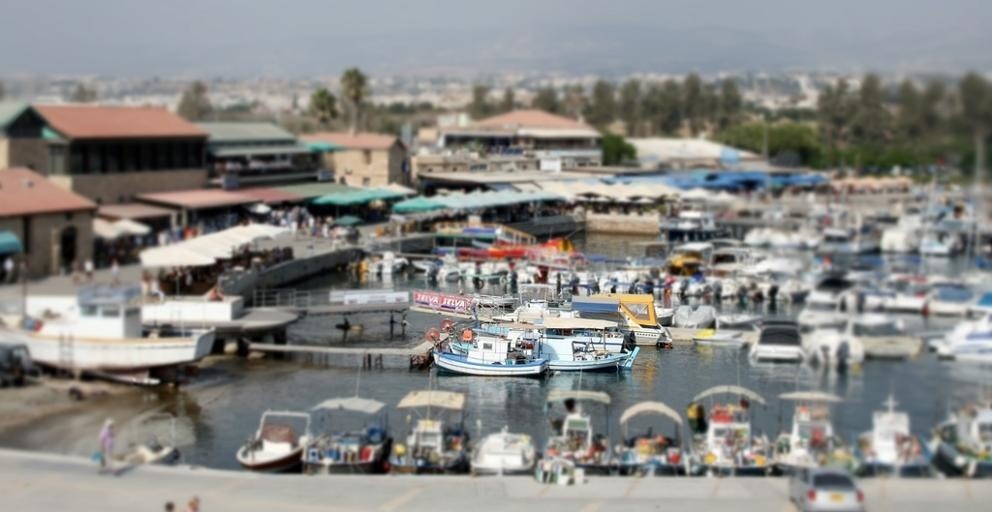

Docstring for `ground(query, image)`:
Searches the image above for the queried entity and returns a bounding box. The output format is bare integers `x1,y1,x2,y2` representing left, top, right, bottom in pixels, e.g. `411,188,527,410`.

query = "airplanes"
745,308,924,372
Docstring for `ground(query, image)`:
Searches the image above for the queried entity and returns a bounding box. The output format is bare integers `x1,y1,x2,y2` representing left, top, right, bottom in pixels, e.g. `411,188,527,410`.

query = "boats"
687,384,775,474
931,388,992,478
235,410,315,467
470,432,537,473
301,397,392,473
830,169,985,256
491,299,635,339
390,390,470,474
933,318,992,364
365,253,513,288
933,312,990,364
541,328,640,371
548,334,631,369
670,305,763,350
541,390,613,478
856,397,936,467
107,446,182,466
0,283,216,376
580,293,673,346
0,291,216,370
432,332,549,375
660,207,838,294
140,289,305,330
773,392,860,473
431,316,547,379
617,399,686,475
809,268,992,322
520,257,682,296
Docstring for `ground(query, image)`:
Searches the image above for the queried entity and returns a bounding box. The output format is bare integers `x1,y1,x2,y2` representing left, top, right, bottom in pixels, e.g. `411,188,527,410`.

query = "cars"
791,467,868,512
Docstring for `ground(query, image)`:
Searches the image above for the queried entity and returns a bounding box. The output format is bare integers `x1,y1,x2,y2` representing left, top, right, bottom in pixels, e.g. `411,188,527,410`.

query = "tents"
776,386,848,414
691,382,770,420
619,399,687,440
308,395,388,434
397,386,467,430
541,389,616,424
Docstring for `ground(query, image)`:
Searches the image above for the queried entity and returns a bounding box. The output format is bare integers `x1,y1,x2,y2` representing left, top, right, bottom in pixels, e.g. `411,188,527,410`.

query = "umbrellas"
393,194,451,210
645,165,829,193
379,181,418,193
313,191,370,208
306,140,348,158
365,187,406,200
332,214,364,226
